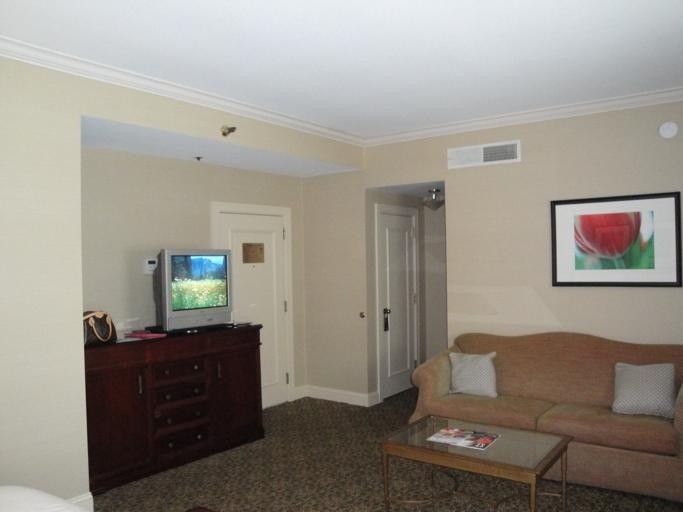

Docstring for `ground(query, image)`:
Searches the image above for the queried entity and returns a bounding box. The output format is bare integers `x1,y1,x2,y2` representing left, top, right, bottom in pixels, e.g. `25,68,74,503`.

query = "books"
123,331,167,340
426,425,502,451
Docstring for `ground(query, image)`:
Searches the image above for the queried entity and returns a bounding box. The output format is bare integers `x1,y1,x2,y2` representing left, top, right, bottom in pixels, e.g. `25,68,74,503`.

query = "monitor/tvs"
152,248,231,331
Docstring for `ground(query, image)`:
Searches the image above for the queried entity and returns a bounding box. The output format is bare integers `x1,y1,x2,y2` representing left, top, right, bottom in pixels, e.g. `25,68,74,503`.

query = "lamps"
419,184,445,213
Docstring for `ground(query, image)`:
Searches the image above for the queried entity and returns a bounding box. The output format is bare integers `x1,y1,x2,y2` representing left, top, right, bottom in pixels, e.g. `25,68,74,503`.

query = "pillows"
445,350,499,399
612,361,678,419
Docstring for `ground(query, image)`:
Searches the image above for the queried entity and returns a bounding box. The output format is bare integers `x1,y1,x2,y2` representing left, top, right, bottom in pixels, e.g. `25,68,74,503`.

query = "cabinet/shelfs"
83,320,268,499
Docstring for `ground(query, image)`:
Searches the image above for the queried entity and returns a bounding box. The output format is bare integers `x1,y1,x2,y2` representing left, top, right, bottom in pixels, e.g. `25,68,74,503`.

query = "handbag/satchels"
82,308,118,347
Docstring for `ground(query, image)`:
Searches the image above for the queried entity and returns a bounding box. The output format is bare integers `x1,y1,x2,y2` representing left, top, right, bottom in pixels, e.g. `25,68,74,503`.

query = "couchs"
406,329,682,501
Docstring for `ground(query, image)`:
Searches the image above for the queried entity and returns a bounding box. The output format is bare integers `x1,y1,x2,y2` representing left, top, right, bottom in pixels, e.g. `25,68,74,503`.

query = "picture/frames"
550,190,683,289
